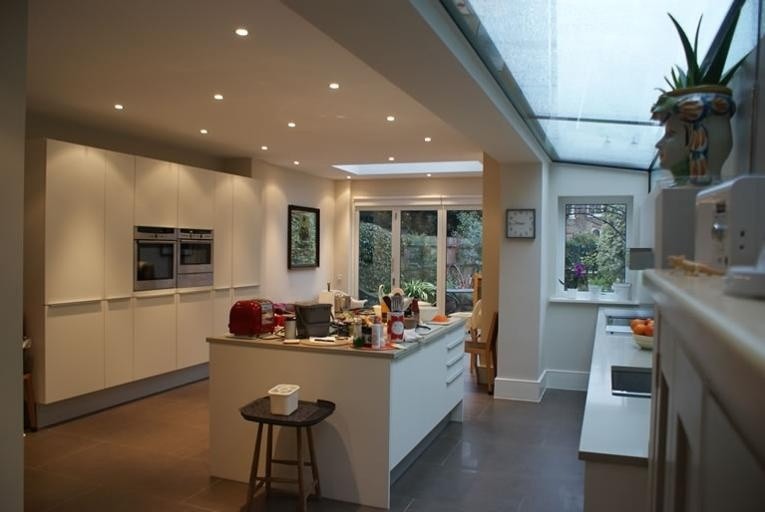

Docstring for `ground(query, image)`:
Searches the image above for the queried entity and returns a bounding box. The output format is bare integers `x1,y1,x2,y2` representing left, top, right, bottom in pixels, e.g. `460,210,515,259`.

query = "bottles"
372,317,382,351
410,295,422,326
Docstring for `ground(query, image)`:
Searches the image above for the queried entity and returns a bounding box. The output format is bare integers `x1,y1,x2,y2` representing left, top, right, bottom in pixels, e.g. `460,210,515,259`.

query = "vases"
577,277,588,291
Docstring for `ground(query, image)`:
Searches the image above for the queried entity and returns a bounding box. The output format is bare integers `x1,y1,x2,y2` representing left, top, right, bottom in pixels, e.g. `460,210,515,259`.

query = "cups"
387,311,415,343
283,317,297,341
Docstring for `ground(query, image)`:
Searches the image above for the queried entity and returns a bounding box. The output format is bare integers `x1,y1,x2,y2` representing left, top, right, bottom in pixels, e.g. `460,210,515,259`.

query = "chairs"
464,311,498,395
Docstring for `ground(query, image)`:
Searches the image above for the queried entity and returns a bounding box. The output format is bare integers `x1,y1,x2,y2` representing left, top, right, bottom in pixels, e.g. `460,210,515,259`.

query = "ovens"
134,226,212,292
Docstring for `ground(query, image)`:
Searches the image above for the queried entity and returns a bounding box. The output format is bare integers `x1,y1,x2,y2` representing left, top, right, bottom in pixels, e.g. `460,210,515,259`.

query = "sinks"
610,364,653,398
604,309,655,335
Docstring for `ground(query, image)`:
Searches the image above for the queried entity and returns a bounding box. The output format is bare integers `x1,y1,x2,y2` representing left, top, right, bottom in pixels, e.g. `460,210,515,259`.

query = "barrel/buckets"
292,303,335,338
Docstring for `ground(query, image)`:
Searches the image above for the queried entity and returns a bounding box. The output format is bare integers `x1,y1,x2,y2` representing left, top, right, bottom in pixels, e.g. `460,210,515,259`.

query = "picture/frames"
287,203,321,269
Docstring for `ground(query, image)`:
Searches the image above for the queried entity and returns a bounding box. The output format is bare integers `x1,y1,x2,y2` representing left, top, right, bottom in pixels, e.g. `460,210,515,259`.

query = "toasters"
228,299,275,338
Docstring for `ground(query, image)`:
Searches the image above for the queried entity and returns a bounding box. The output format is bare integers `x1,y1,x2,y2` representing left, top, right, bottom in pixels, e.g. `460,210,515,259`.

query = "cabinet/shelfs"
212,172,262,289
135,157,216,232
45,141,106,303
105,152,136,299
446,326,466,412
46,287,259,404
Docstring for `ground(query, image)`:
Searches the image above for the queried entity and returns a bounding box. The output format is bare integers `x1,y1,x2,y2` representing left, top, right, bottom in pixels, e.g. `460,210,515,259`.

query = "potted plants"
646,0,749,197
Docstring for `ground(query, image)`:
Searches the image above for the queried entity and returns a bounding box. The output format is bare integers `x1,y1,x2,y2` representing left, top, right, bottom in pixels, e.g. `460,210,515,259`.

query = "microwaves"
695,176,764,270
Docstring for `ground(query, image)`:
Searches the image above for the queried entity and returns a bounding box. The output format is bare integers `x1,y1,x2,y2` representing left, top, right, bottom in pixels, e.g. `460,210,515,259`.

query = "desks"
238,395,336,512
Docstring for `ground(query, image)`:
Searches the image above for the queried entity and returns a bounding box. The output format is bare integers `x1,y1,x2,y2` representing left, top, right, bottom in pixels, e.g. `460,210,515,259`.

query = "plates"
425,317,460,325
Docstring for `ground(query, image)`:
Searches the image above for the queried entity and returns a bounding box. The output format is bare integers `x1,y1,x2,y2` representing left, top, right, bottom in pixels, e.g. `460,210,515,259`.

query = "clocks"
503,207,537,240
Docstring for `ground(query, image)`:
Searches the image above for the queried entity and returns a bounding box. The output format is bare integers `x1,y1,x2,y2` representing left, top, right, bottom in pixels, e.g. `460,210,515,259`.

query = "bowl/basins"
630,335,656,349
723,266,765,299
418,307,439,322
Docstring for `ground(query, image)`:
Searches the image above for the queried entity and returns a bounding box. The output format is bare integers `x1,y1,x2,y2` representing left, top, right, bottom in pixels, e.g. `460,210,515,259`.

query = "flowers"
575,263,589,279
